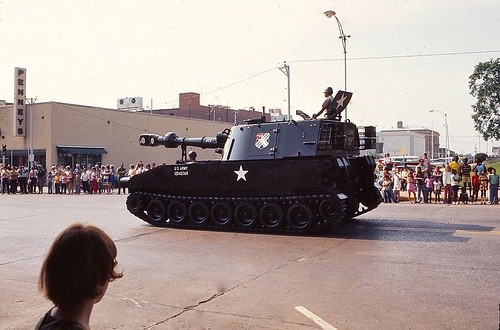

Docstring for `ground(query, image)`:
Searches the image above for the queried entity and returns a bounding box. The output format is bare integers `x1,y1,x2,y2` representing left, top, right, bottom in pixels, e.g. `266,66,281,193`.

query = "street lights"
429,109,449,163
324,10,351,122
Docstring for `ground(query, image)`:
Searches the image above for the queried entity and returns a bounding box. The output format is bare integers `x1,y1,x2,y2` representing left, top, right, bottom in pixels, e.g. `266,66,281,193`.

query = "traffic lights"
2,144,7,155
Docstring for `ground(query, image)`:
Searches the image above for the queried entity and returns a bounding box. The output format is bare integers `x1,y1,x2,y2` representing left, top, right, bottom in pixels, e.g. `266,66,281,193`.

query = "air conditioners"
117,97,142,112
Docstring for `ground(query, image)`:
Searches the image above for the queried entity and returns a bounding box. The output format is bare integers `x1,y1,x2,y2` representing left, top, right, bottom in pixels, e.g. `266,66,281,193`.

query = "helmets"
323,87,333,93
188,151,197,156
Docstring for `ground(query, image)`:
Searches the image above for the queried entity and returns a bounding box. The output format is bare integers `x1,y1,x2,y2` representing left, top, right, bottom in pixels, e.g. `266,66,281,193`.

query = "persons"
374,152,499,205
188,151,198,161
0,161,165,196
313,87,335,119
34,224,123,330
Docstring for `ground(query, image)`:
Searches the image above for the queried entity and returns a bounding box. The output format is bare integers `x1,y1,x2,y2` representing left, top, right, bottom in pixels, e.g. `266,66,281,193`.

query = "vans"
386,154,457,166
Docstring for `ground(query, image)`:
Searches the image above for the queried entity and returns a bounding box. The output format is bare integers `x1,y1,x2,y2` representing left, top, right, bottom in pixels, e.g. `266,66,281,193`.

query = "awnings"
1,149,45,156
57,148,107,154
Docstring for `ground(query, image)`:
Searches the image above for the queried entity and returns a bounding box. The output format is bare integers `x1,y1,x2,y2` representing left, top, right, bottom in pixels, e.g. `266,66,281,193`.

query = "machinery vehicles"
124,89,386,235
